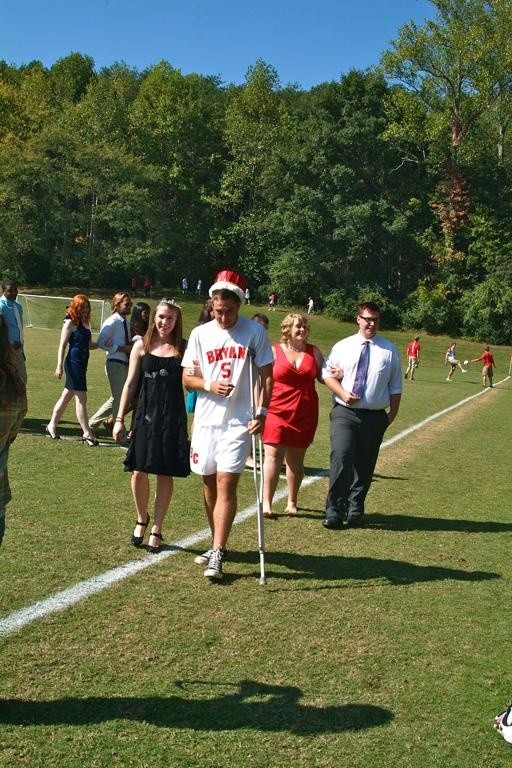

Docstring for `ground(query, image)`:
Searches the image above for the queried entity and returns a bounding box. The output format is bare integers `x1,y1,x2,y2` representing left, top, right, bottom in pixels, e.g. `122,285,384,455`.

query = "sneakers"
196,549,228,580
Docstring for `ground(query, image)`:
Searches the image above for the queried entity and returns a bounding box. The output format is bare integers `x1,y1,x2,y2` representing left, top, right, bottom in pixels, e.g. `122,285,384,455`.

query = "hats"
209,269,247,305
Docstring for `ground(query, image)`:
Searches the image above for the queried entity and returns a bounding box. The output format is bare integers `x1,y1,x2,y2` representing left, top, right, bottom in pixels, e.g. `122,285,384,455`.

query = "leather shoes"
131,512,150,545
323,517,359,529
146,534,163,554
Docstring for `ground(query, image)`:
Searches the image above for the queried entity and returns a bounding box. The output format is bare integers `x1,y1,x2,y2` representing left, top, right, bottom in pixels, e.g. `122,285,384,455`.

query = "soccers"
463,359,469,365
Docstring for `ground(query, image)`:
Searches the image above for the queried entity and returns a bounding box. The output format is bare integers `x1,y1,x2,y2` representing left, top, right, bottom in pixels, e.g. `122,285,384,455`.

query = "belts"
106,358,129,365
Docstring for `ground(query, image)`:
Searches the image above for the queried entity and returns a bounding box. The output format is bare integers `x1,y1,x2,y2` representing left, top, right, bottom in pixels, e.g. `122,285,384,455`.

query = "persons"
43,293,114,448
267,292,278,312
181,275,188,295
319,301,403,531
178,269,276,583
184,297,215,436
403,335,421,380
129,278,138,297
88,290,137,441
105,297,202,555
244,450,263,471
1,278,30,418
244,288,252,305
444,341,469,382
307,296,317,314
129,301,151,340
0,313,30,548
196,277,202,296
470,344,497,388
251,312,272,330
142,277,153,297
260,311,344,518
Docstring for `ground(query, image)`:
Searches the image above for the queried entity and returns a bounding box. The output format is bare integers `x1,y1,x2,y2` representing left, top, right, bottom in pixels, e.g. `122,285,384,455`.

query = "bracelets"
255,406,268,416
114,416,125,423
204,378,216,392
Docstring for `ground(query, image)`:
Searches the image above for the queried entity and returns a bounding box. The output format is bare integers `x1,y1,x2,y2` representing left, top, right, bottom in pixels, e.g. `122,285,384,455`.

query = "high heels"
82,435,99,446
44,426,61,439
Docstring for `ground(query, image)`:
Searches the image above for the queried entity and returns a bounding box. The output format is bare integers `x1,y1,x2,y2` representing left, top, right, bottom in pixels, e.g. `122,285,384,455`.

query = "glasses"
359,314,379,322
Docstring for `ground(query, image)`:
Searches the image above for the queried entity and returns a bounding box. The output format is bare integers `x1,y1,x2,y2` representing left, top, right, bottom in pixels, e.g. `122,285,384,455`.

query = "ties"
12,303,23,344
352,343,371,401
124,321,129,345
